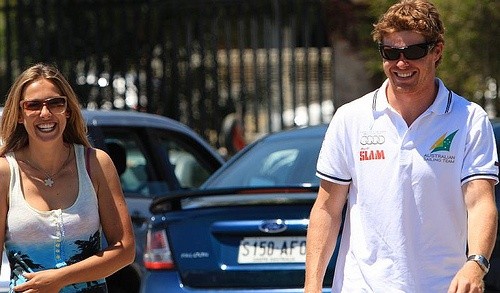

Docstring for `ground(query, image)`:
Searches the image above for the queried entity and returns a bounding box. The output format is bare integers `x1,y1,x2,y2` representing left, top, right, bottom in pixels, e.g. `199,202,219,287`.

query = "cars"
466,119,500,293
140,123,350,293
78,109,225,293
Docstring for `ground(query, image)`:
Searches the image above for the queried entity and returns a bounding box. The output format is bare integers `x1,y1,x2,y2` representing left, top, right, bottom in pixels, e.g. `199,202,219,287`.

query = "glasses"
20,96,68,114
379,36,437,60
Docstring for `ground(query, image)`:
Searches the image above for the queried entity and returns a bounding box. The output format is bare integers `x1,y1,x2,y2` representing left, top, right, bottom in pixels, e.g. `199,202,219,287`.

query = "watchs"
466,254,491,275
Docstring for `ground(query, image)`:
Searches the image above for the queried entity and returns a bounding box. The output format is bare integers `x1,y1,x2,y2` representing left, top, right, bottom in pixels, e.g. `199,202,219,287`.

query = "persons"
0,64,136,293
302,1,500,293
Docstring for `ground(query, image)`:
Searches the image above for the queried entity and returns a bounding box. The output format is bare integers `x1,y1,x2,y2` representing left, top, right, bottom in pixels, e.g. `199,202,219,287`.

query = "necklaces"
21,144,72,187
